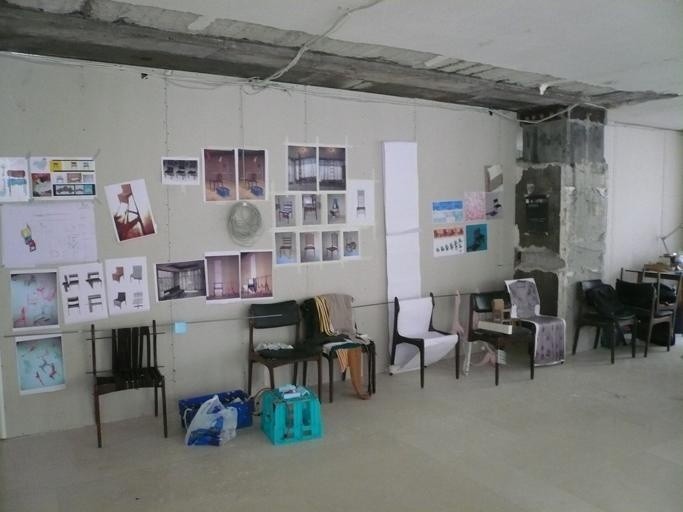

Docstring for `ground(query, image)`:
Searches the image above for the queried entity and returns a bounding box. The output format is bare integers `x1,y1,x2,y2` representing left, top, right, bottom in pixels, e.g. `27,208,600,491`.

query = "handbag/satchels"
586,285,624,316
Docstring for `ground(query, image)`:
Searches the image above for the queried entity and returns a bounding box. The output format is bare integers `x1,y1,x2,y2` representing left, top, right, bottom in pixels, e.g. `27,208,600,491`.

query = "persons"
466,227,485,252
485,197,501,217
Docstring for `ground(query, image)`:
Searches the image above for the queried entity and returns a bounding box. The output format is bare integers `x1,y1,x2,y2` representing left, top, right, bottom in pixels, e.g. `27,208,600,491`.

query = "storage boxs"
177,389,254,433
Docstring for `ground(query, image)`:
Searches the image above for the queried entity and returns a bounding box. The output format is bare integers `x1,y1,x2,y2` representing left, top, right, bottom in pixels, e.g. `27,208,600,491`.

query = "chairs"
464,290,534,387
571,267,677,364
85,320,167,449
504,277,565,369
389,292,460,389
246,294,377,404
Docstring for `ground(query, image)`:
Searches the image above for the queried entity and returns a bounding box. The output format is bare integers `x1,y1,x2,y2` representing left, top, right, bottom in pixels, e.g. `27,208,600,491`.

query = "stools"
260,386,322,446
